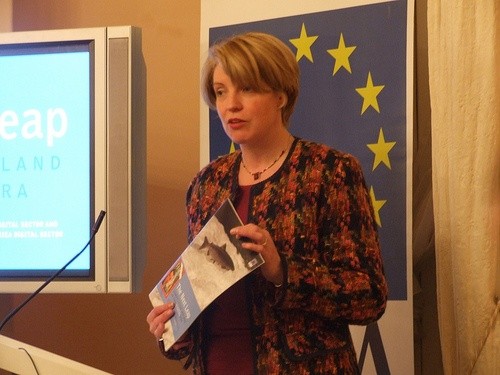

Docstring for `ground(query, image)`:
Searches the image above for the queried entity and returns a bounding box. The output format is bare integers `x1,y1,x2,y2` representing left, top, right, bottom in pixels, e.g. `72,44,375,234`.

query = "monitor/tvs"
0,25,136,294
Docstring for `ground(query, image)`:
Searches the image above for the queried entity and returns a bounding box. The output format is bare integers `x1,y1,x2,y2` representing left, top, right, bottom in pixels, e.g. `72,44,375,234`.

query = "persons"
146,30,388,374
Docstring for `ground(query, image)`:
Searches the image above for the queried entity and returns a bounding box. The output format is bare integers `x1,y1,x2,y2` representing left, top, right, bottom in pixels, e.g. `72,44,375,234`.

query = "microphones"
0,211,107,331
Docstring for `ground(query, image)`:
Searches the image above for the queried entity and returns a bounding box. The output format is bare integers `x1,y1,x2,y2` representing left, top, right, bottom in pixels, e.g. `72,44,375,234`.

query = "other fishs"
199,236,235,272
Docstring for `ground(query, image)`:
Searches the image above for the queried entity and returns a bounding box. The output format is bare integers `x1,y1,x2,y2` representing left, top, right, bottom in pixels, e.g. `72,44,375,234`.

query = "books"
147,196,265,353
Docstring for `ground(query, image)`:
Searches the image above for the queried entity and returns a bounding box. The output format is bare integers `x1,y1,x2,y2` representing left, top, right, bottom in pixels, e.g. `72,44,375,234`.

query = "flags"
209,0,411,301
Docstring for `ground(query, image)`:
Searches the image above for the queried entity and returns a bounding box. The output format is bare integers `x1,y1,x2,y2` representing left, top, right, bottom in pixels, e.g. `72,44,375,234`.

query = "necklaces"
240,133,293,182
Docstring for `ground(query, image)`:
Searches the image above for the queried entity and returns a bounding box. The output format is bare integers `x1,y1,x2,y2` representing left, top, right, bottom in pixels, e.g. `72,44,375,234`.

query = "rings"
262,240,268,247
